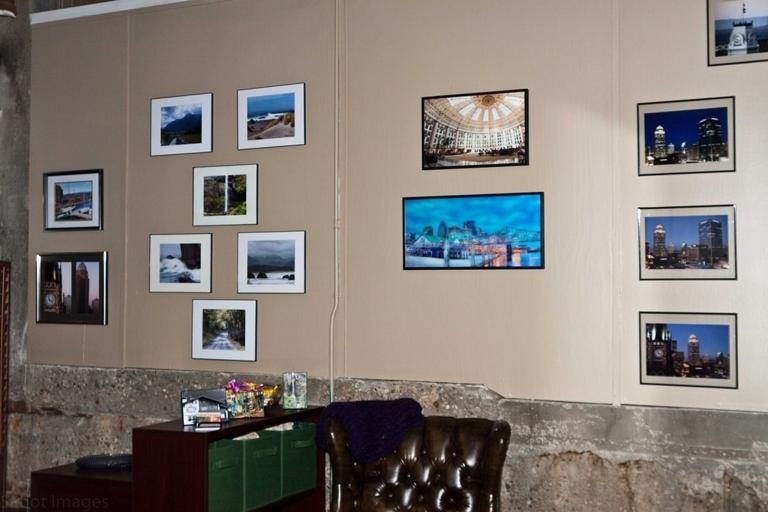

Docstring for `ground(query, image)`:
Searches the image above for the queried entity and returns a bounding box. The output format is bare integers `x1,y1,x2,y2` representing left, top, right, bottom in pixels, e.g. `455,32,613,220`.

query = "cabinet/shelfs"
28,462,132,512
132,404,323,512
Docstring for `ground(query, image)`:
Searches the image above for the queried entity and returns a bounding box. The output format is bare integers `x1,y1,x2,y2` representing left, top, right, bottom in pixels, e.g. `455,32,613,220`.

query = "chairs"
322,395,423,511
354,397,510,512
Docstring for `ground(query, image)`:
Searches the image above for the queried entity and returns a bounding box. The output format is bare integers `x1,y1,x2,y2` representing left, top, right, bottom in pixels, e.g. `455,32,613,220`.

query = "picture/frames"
191,298,256,360
705,1,768,67
636,95,736,177
237,229,306,294
42,168,103,232
402,192,544,269
149,233,212,293
237,83,306,151
639,311,738,390
150,92,214,157
637,203,738,281
192,163,258,227
36,250,108,325
421,88,529,170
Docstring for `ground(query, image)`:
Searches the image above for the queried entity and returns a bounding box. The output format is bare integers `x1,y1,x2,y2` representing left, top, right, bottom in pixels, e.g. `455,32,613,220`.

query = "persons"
474,141,525,163
